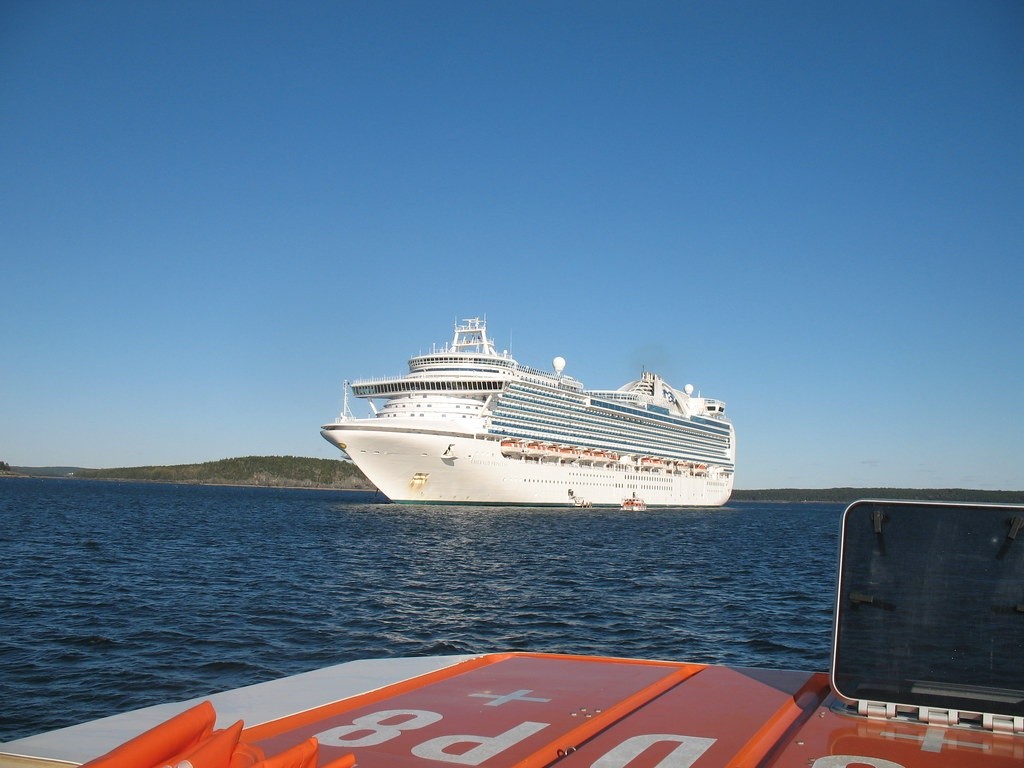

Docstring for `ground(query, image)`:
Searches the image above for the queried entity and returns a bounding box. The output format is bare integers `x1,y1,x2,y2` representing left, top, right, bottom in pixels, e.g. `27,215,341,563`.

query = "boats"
501,439,526,457
619,491,647,513
640,455,708,476
319,312,737,505
544,446,562,458
560,448,579,462
579,446,608,463
524,440,548,458
